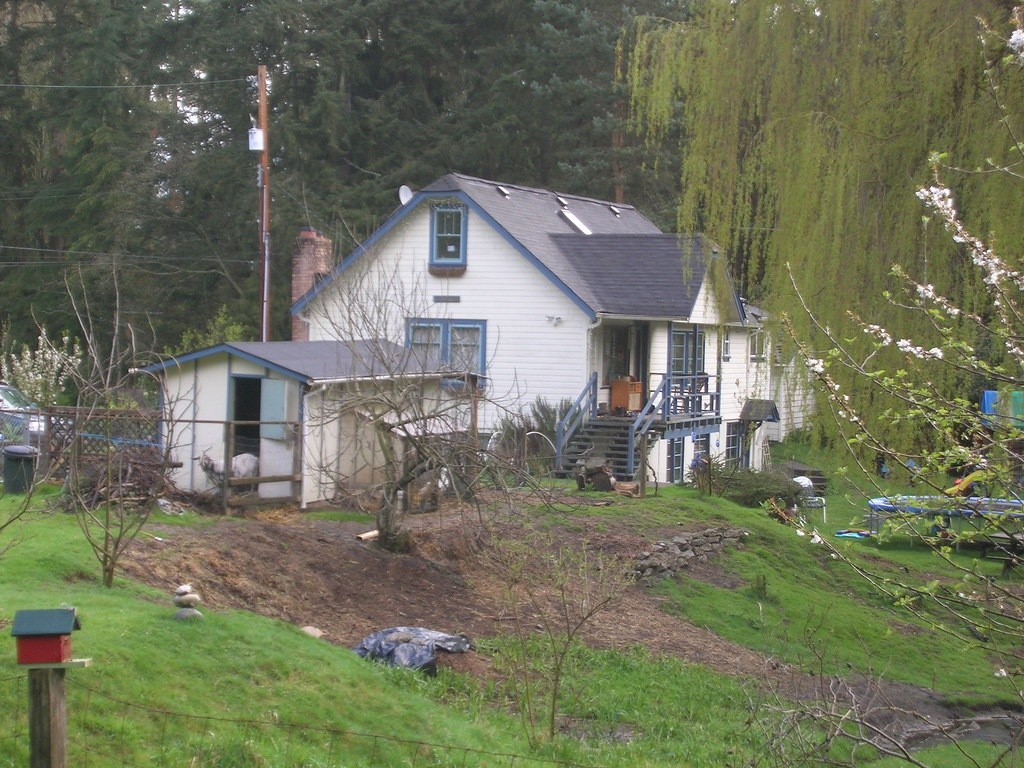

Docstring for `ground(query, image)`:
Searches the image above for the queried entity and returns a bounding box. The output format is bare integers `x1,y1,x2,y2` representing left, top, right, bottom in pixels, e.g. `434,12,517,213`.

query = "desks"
611,374,641,416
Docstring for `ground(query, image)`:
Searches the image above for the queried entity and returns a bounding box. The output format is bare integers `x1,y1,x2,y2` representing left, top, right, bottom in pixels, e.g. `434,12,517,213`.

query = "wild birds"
414,467,450,504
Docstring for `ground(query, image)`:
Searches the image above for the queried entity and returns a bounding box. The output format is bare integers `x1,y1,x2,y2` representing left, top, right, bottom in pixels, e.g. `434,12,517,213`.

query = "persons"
906,454,921,487
875,450,884,476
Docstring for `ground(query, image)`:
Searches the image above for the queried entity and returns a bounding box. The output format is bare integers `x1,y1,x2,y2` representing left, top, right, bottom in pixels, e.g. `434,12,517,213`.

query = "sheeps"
192,446,260,492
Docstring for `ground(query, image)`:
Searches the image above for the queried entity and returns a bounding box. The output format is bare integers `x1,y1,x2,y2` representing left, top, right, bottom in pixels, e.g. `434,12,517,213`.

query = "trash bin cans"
2,446,42,494
